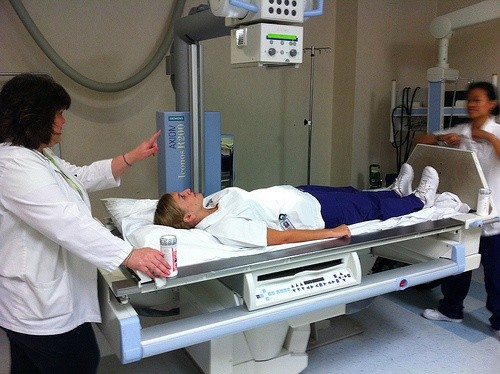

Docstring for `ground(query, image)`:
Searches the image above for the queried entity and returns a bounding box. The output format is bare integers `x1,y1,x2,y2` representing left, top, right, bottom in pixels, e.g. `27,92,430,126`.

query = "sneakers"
391,163,414,198
412,166,440,209
423,308,464,322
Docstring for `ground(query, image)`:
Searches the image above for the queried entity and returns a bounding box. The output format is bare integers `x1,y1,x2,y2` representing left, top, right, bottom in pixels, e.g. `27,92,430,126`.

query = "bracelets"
122,153,133,166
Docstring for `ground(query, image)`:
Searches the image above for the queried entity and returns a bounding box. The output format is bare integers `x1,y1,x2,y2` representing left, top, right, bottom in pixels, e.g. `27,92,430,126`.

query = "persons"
0,73,171,374
153,163,439,248
414,81,500,340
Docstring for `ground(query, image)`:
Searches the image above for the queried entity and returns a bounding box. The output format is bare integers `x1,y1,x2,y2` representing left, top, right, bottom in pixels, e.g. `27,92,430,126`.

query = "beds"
98,144,500,374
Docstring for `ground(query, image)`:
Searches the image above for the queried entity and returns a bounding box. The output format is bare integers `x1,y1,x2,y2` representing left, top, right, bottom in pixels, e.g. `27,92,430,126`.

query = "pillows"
101,197,230,264
